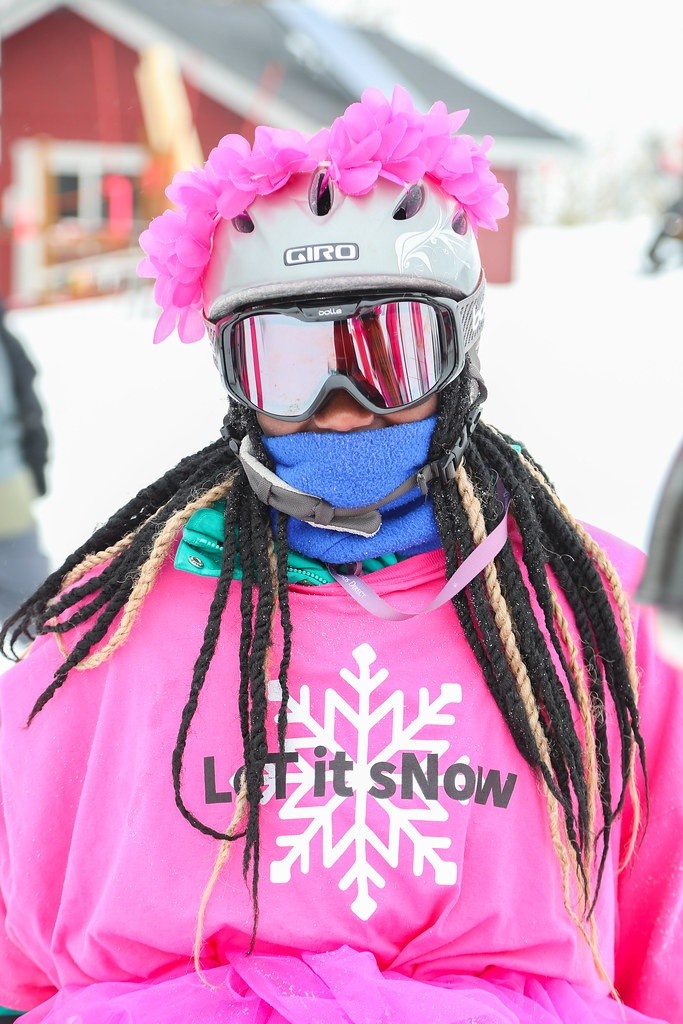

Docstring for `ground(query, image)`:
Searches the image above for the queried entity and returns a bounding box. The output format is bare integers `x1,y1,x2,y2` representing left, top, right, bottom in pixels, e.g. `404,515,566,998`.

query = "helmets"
137,84,509,344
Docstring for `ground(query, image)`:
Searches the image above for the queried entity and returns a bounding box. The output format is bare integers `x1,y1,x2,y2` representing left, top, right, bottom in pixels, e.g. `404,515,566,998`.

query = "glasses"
215,291,460,423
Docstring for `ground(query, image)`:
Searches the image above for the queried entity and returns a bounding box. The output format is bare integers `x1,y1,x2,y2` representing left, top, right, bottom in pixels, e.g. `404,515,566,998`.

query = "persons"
0,85,683,1024
0,316,62,629
